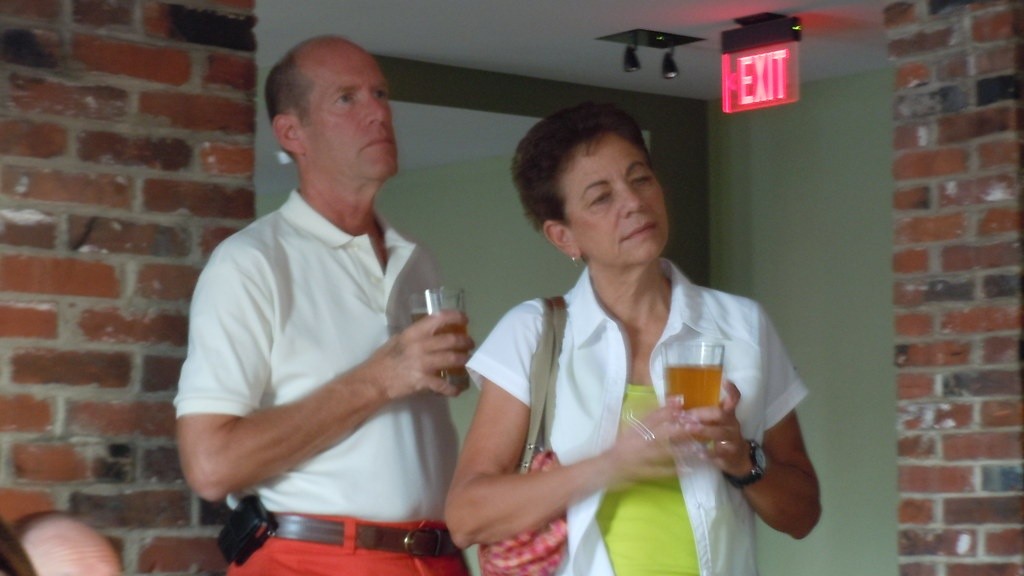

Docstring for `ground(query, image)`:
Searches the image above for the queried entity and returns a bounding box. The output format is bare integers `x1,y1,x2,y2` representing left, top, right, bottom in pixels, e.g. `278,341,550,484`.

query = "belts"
269,514,462,556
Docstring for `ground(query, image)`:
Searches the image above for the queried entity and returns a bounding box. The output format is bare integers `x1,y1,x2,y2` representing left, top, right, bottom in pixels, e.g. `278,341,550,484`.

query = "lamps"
624,42,641,72
663,43,679,79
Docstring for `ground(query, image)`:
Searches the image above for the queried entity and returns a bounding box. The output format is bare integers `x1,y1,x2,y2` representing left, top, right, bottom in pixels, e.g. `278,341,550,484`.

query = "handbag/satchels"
477,296,568,576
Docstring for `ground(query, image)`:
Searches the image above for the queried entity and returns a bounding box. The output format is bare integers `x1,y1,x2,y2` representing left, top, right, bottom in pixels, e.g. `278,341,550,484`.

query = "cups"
659,341,724,451
409,286,470,393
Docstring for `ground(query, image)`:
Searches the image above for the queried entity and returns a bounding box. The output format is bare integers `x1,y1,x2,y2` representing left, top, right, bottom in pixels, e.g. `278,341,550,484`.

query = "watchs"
723,440,766,488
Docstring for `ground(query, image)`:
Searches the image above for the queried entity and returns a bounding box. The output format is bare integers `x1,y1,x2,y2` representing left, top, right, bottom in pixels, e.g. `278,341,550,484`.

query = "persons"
1,513,122,576
442,100,821,576
173,37,471,576
